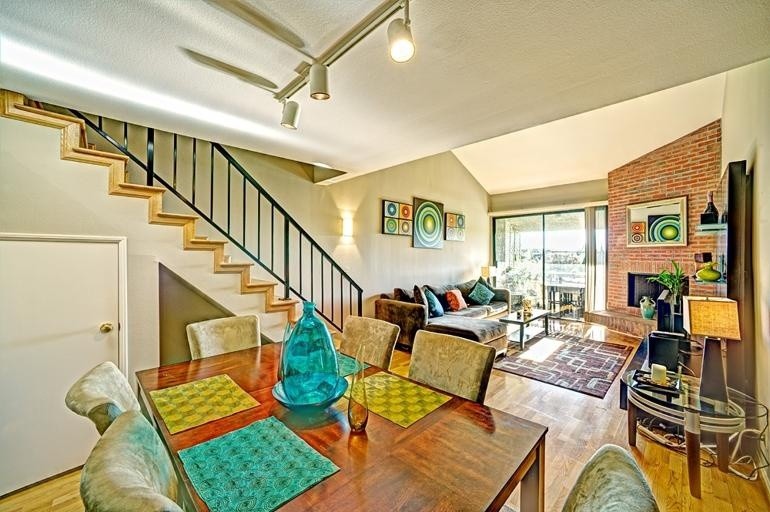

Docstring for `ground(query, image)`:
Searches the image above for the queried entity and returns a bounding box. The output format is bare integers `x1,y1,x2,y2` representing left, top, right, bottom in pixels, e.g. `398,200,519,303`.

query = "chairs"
407,331,495,406
338,315,400,371
64,360,158,437
79,409,203,511
464,276,497,306
186,314,262,360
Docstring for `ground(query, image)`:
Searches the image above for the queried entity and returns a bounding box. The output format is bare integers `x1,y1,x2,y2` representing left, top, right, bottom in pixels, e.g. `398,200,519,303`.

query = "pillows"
468,282,495,306
445,290,467,312
424,287,444,317
413,284,428,309
394,285,443,308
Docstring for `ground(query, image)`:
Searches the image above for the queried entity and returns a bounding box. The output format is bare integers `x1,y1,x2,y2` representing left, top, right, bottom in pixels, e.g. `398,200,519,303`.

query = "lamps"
309,64,331,99
482,266,497,286
280,97,301,130
683,295,741,403
387,0,415,64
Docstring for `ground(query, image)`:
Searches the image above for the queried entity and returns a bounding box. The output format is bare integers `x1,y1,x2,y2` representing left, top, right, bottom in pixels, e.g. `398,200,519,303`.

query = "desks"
547,284,586,317
620,336,691,411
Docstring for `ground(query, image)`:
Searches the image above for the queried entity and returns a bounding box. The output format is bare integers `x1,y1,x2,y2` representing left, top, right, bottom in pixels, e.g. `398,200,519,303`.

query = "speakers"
648,333,679,371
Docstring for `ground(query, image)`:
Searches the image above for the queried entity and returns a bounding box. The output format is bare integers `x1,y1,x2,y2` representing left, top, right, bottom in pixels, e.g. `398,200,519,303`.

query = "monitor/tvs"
657,289,669,331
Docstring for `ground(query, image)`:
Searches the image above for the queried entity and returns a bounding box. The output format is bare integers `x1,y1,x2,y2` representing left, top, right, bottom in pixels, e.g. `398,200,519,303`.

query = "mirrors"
626,196,687,248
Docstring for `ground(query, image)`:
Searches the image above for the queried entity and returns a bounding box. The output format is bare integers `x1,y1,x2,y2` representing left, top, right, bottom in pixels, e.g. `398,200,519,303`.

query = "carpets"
493,328,634,399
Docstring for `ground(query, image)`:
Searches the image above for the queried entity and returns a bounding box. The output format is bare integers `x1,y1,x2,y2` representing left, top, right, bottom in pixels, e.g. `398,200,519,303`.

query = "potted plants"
645,261,690,315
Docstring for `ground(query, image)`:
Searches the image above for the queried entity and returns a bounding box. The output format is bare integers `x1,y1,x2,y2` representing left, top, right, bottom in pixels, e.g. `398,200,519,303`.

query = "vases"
277,321,291,382
639,296,656,321
349,344,368,433
695,261,722,283
282,301,340,405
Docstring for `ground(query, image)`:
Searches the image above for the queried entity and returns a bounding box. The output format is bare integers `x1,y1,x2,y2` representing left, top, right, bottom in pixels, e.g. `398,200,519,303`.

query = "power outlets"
761,426,768,447
637,423,671,446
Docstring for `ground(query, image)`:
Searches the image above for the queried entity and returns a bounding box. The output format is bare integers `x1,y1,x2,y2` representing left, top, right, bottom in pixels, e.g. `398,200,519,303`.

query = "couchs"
374,287,511,361
